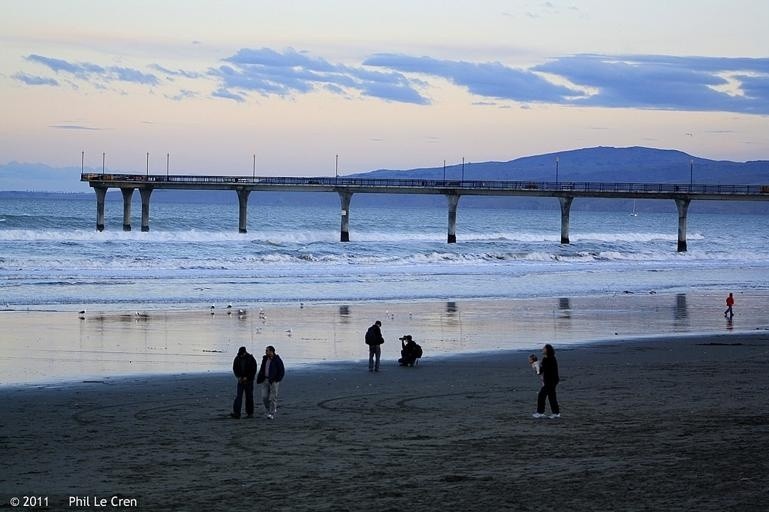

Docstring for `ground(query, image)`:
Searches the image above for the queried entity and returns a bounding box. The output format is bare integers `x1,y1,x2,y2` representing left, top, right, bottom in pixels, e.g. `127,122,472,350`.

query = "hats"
237,346,247,356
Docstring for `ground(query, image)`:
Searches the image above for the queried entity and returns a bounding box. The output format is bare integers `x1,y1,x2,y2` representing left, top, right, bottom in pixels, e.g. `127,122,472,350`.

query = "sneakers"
265,412,276,420
232,412,253,419
533,413,561,419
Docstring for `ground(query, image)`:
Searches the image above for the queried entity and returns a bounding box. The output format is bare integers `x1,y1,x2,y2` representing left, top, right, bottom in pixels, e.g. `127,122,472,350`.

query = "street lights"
147,152,148,175
167,153,169,175
556,156,560,189
444,160,445,187
462,157,464,188
254,152,255,176
691,160,693,192
82,150,84,174
103,152,105,174
336,155,338,178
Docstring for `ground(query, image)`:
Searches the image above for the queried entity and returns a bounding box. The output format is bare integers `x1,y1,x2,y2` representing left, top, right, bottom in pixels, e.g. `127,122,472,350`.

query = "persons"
398,334,422,368
230,345,285,420
724,293,735,316
365,321,385,371
527,343,562,419
